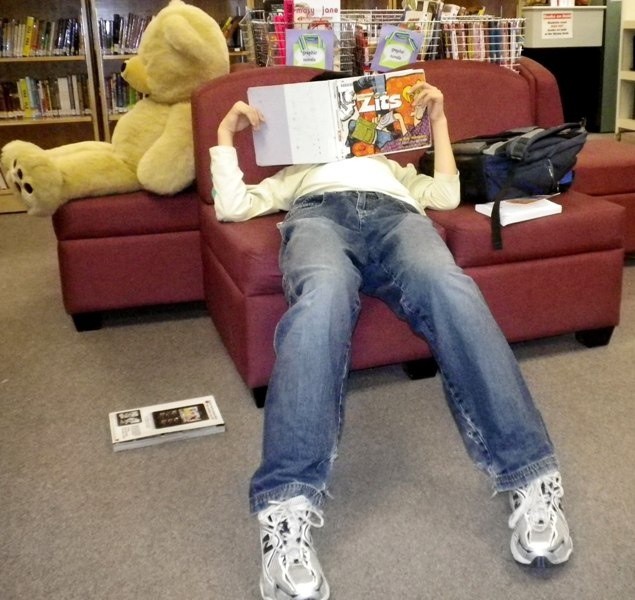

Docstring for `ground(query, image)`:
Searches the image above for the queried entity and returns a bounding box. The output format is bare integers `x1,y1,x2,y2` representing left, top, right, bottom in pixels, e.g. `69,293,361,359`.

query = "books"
220,15,242,41
0,15,82,58
474,192,564,228
108,395,226,452
0,72,92,119
357,1,526,74
104,72,147,115
247,69,433,167
97,12,152,55
247,0,354,72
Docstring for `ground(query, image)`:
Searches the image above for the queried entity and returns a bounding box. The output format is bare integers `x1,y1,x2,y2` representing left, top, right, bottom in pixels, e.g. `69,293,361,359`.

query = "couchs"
192,68,447,408
52,61,260,331
523,53,635,258
386,58,628,346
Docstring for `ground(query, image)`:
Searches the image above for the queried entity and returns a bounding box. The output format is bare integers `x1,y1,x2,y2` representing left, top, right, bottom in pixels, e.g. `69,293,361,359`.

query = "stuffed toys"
0,0,230,218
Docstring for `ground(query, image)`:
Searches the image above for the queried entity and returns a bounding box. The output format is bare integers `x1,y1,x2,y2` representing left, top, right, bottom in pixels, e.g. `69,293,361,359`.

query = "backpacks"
419,118,588,250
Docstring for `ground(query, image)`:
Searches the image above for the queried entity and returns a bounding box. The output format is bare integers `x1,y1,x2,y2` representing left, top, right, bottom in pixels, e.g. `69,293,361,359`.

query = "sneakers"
508,471,573,568
257,494,330,600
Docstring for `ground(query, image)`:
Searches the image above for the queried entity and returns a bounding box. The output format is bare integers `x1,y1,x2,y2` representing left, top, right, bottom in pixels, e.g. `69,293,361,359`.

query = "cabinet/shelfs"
92,2,247,144
0,0,100,215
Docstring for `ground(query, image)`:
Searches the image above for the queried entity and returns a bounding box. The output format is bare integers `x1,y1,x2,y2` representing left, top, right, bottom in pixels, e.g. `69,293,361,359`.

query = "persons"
209,71,574,600
